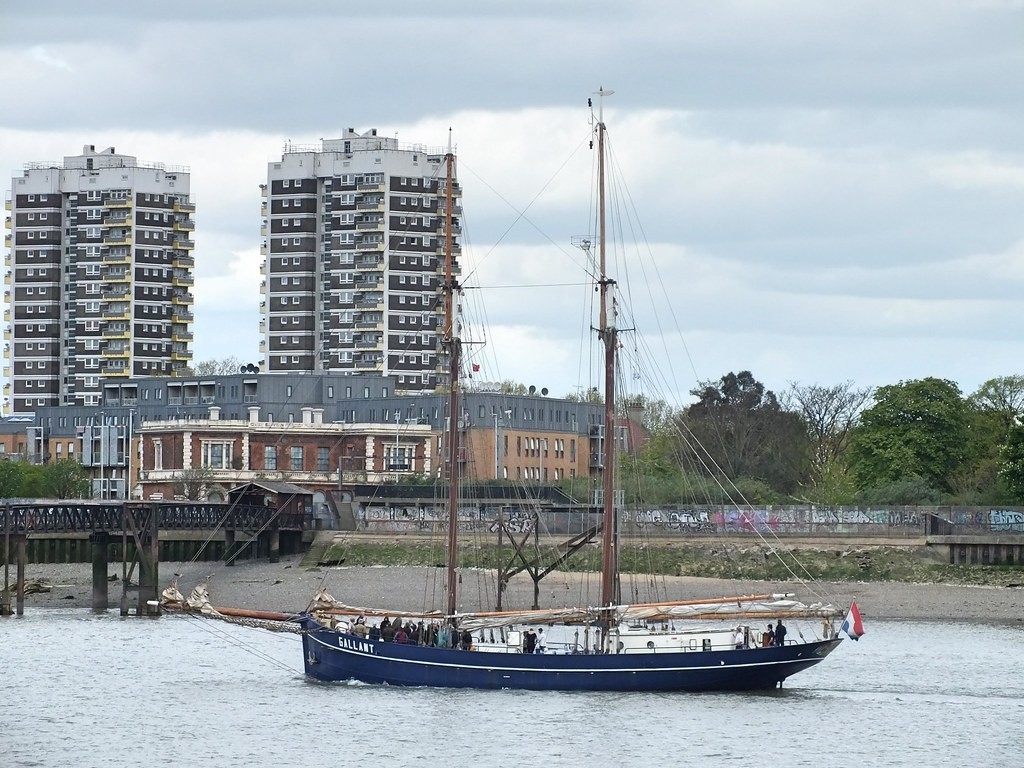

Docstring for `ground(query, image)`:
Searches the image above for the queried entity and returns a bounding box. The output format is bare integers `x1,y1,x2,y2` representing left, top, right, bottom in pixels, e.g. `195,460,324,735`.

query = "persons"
348,611,787,654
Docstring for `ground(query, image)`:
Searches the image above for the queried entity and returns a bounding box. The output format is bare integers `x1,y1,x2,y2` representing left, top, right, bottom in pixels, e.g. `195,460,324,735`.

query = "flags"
841,601,865,642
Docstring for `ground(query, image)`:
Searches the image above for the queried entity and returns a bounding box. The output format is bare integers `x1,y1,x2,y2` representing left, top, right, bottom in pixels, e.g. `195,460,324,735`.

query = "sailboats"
149,87,863,694
